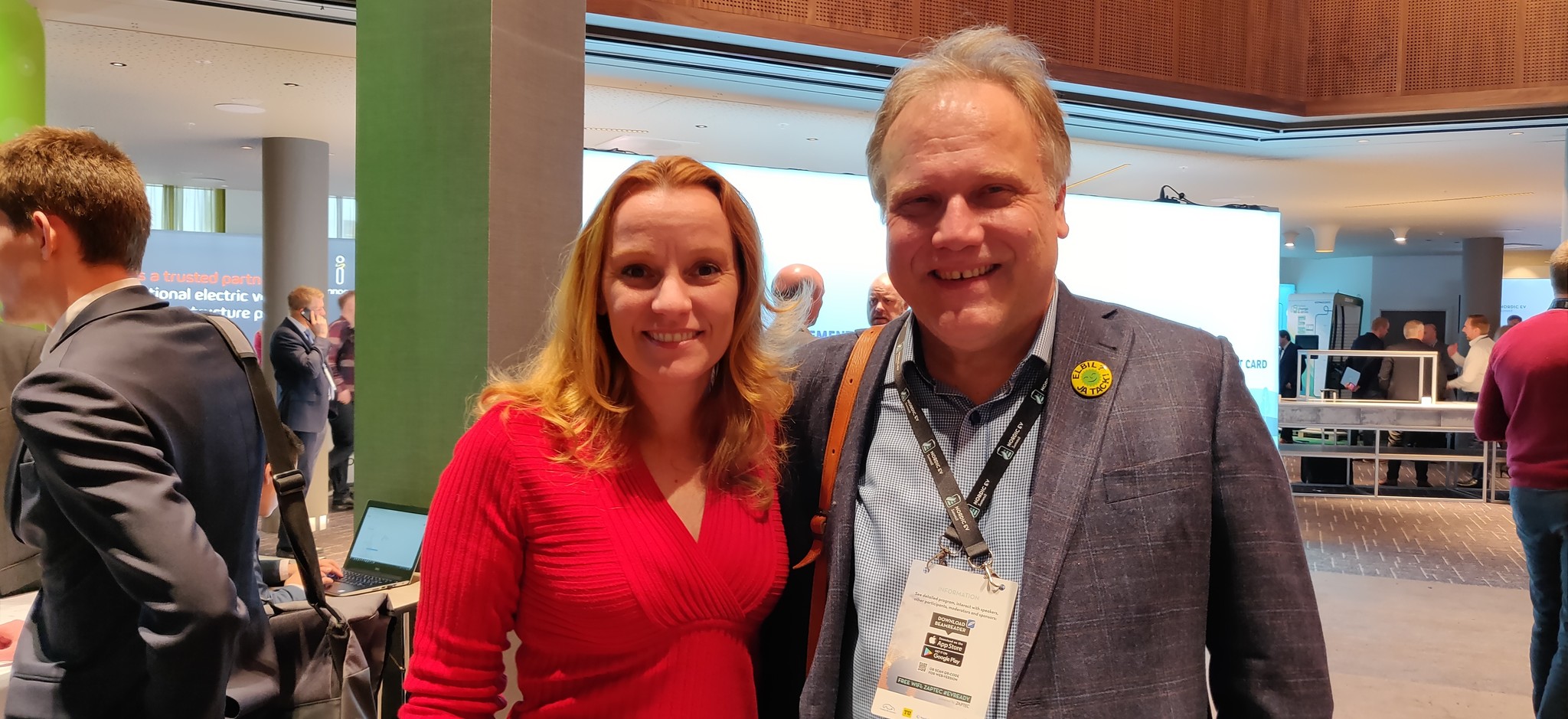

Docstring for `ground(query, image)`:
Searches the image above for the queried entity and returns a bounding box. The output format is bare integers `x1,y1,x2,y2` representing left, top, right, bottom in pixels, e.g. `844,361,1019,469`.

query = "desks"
327,578,421,719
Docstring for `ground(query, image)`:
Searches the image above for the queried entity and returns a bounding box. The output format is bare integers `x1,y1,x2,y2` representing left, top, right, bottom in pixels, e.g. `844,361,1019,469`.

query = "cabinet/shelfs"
1278,349,1510,504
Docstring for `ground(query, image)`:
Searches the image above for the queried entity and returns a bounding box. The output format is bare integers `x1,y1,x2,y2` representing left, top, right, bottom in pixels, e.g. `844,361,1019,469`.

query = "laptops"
322,499,430,597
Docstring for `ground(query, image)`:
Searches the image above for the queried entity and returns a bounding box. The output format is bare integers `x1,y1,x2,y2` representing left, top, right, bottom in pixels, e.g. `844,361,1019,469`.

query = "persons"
327,290,355,511
1473,240,1568,719
1279,330,1306,443
751,25,1333,719
1507,315,1522,327
270,285,336,556
1377,320,1447,487
397,153,790,719
1446,314,1496,488
1342,317,1390,463
867,273,909,326
765,264,825,354
1422,323,1455,375
254,422,343,605
0,125,267,719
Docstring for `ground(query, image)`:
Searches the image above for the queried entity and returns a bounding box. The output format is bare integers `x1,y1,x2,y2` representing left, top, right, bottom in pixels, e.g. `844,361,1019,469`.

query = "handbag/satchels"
261,591,396,719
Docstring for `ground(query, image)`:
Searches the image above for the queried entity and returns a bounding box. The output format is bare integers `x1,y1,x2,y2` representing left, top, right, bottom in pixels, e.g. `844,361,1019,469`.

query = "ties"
304,329,335,401
1279,348,1284,361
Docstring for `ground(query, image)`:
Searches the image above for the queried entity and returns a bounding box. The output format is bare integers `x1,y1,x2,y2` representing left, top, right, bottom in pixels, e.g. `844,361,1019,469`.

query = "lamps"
1283,231,1299,247
1391,227,1410,242
1312,227,1339,252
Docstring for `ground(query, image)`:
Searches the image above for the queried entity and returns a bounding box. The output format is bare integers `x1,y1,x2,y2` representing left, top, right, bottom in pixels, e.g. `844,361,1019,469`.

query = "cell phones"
301,307,311,322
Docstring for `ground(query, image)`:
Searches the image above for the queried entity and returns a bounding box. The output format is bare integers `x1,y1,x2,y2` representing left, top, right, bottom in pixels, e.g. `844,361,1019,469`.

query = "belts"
1465,392,1479,394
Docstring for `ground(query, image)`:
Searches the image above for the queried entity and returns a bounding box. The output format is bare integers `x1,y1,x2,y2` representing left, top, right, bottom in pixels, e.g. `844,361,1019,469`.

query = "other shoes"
331,490,354,510
1378,479,1397,486
276,539,326,560
1362,458,1387,463
1279,437,1293,443
1417,480,1433,487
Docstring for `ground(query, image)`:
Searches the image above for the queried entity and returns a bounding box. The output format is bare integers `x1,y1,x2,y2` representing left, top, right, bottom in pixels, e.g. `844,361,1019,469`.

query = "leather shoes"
1487,480,1489,488
1457,478,1482,488
